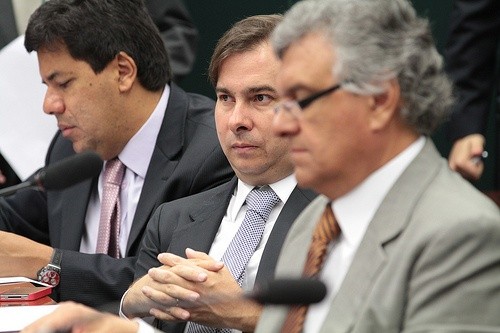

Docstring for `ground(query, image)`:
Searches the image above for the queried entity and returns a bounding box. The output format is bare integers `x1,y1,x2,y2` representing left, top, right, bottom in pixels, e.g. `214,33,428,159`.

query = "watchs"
34,245,62,287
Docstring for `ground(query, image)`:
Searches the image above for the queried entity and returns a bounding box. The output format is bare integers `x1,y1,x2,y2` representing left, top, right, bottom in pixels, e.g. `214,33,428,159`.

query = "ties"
279,203,341,333
184,187,282,333
95,159,125,259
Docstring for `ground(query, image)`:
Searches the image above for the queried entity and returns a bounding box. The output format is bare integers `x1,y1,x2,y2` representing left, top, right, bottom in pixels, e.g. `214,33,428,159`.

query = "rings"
177,298,180,307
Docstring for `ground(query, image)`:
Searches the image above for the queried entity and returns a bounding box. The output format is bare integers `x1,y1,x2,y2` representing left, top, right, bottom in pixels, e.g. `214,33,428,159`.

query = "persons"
1,0,236,314
140,0,500,188
18,0,500,333
116,12,321,333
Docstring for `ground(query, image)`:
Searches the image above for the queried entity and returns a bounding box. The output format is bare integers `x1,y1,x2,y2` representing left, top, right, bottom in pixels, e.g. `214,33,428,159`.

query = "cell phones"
0,287,50,301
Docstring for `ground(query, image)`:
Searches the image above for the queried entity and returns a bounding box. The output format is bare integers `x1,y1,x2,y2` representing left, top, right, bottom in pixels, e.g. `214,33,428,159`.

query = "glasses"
274,83,341,112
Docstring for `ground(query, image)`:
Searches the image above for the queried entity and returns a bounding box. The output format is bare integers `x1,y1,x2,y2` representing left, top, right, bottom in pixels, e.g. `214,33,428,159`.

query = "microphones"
95,277,328,316
0,152,103,196
